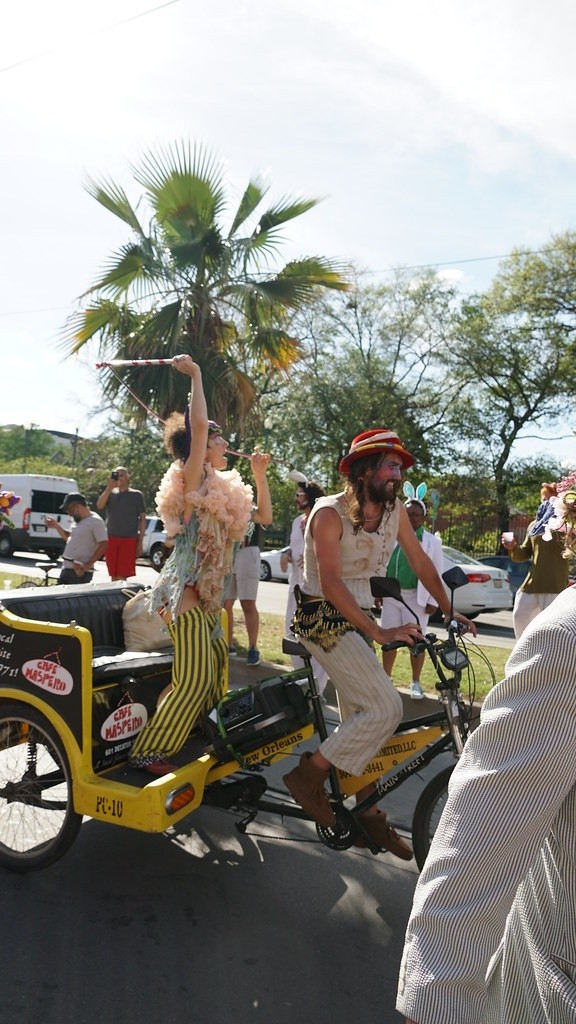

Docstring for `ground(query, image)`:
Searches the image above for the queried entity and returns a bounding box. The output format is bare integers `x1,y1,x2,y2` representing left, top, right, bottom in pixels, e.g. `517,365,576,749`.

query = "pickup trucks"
98,516,167,566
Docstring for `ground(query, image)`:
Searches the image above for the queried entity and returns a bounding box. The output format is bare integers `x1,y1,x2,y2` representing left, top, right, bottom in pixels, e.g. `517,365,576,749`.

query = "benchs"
0,582,206,775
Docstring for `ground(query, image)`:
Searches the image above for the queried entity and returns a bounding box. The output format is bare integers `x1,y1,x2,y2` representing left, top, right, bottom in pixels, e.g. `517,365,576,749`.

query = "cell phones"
111,472,119,481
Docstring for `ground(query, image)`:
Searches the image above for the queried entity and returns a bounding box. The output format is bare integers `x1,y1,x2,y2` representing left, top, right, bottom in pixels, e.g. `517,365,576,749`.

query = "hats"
340,430,416,477
59,492,87,511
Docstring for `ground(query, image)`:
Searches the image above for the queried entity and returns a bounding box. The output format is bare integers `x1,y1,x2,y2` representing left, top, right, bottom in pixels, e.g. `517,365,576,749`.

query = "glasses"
296,492,306,498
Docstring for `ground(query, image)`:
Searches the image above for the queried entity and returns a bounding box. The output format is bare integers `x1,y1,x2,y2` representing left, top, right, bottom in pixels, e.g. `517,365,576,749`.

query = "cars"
428,545,513,623
258,543,292,581
475,556,534,612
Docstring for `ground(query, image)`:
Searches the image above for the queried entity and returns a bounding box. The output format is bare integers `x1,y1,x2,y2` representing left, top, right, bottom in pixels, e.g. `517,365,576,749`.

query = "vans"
0,473,80,559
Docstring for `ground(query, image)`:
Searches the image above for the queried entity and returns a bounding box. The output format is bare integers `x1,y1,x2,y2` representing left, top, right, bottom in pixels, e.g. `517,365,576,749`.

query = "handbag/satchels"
121,589,174,651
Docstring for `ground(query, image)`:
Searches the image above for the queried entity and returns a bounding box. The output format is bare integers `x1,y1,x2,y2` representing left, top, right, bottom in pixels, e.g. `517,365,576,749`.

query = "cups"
503,532,514,543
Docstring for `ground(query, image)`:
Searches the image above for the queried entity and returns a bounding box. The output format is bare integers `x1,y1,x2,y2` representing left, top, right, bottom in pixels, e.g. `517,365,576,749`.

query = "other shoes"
145,760,179,774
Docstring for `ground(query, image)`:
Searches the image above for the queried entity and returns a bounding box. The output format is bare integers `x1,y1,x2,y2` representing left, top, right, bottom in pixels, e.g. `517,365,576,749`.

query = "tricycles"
1,566,496,877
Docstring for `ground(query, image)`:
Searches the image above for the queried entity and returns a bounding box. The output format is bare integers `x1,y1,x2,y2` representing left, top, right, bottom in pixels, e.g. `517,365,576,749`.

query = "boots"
355,809,413,862
282,749,336,827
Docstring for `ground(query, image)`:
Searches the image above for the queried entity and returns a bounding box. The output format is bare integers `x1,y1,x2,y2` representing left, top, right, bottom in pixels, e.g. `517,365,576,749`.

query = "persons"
501,482,570,642
394,580,576,1024
374,481,442,699
280,469,329,694
282,430,477,861
44,492,108,586
128,354,272,776
223,522,261,665
97,466,147,582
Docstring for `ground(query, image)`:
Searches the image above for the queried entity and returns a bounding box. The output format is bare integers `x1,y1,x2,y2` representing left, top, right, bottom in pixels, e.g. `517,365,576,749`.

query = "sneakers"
246,647,260,665
409,682,423,700
229,647,238,656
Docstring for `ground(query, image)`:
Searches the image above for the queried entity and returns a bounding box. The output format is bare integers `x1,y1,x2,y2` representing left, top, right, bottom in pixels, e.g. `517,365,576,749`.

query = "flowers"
542,471,576,560
